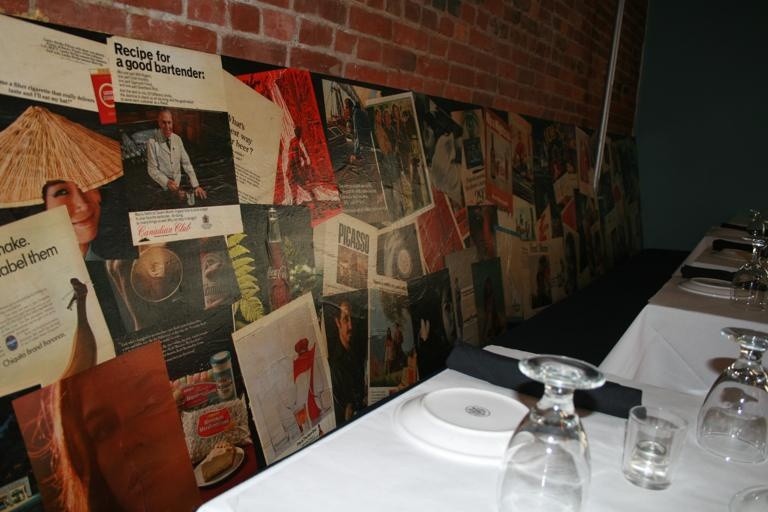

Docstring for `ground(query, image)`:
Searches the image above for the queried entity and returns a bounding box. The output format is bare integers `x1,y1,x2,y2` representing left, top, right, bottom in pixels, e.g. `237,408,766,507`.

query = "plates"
393,385,546,462
677,276,755,300
712,247,755,262
193,446,246,489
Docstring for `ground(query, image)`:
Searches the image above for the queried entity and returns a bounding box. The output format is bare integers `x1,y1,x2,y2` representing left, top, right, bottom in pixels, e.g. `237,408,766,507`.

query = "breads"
200,440,236,483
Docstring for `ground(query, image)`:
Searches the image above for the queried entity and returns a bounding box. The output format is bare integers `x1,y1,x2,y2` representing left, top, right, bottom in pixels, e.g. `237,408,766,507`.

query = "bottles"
265,207,292,313
210,350,238,403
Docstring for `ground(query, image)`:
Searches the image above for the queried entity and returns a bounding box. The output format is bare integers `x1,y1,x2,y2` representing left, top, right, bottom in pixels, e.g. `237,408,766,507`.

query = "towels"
445,340,645,419
719,222,746,231
681,264,768,291
712,240,766,257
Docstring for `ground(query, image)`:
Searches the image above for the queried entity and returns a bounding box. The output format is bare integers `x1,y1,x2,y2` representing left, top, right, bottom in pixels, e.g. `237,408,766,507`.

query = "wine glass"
695,325,768,465
728,237,768,311
495,351,607,512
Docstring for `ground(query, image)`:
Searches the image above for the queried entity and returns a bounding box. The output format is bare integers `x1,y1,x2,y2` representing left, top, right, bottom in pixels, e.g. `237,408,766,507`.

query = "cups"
621,404,688,491
292,403,307,432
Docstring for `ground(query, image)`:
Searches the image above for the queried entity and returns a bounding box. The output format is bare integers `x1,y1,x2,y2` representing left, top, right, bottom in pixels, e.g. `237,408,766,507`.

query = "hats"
0,105,123,208
130,246,184,303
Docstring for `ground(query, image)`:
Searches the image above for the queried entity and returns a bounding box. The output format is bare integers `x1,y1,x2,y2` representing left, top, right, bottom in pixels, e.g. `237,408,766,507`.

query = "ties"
166,137,170,150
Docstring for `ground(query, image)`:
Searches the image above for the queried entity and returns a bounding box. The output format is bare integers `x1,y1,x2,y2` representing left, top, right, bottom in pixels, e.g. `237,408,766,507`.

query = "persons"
416,277,457,378
26,342,202,510
391,104,412,164
383,110,406,171
284,125,327,220
145,110,208,205
383,327,396,376
372,108,401,183
393,322,404,370
331,296,366,426
0,104,126,260
